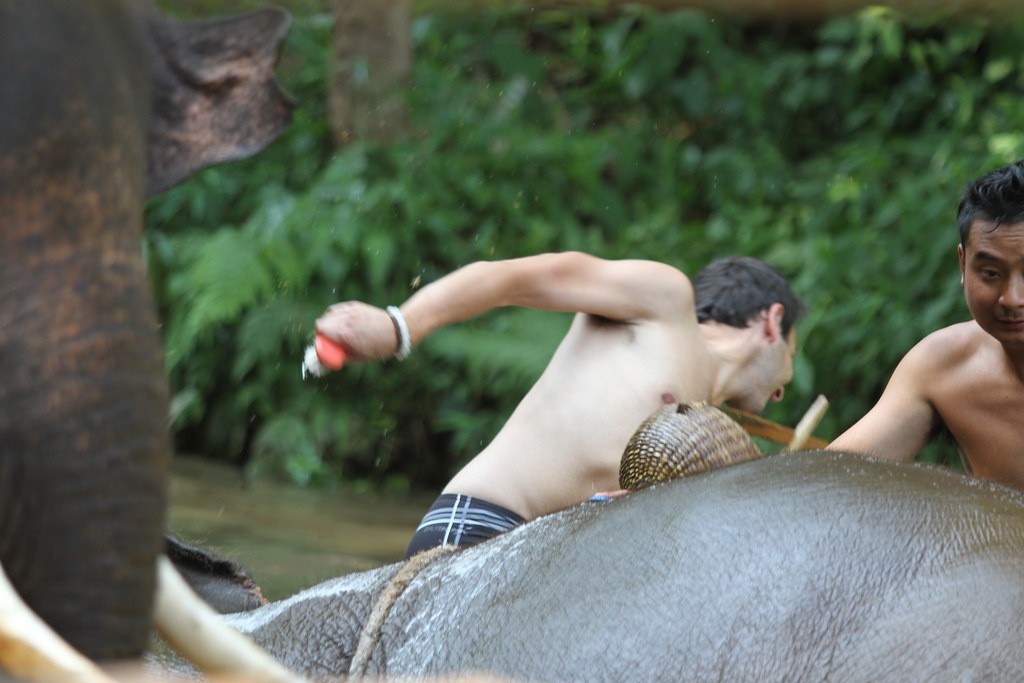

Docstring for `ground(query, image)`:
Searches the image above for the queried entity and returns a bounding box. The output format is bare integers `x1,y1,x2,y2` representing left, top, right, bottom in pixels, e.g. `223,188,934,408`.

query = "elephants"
0,1,1023,683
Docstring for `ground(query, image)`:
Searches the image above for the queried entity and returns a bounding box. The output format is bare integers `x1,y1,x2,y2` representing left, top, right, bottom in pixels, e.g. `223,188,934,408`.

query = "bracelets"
385,303,412,361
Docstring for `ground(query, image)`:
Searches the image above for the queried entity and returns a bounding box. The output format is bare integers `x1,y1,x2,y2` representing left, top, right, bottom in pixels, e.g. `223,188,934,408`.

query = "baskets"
618,397,763,495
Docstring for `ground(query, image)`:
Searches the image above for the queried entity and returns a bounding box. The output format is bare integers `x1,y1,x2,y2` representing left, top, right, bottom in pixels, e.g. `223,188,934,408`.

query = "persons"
593,157,1024,499
315,250,804,563
